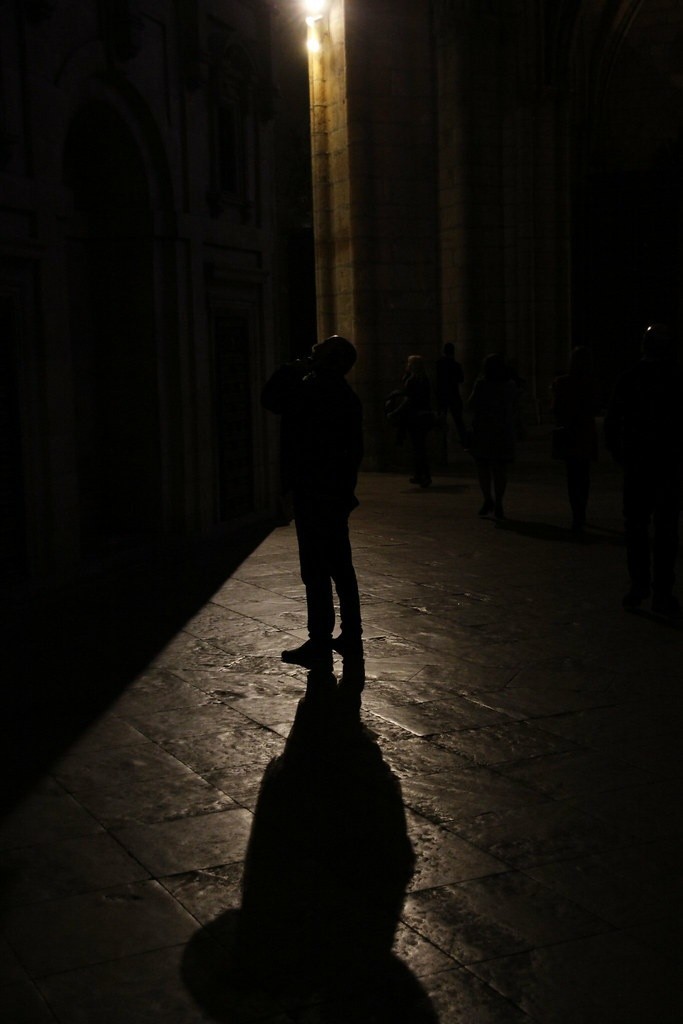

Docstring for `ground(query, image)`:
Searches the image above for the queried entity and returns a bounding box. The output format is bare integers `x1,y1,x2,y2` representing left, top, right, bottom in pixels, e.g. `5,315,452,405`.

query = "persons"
385,343,518,519
603,321,683,612
550,346,599,540
259,334,366,665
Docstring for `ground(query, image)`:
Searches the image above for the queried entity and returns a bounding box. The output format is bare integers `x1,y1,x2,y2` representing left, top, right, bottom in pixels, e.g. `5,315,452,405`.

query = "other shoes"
651,594,683,620
572,510,587,522
478,501,494,516
494,503,507,520
420,478,432,489
621,585,652,609
282,639,333,666
330,628,363,653
409,473,426,485
